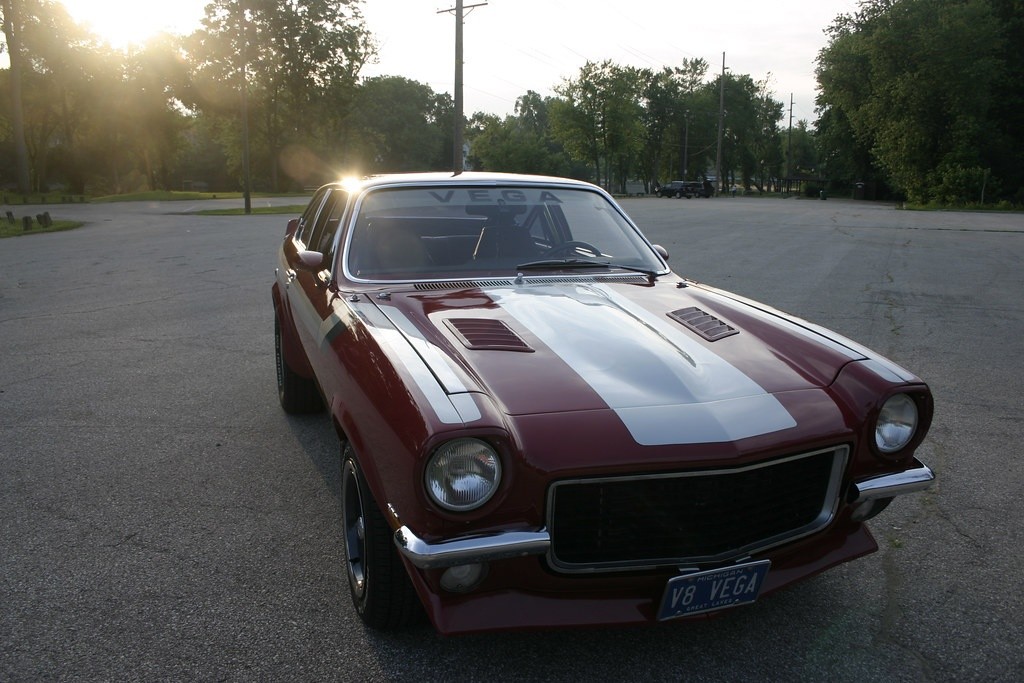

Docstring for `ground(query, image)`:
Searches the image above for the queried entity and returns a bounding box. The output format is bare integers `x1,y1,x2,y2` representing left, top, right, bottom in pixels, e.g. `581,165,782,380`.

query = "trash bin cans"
855,183,865,200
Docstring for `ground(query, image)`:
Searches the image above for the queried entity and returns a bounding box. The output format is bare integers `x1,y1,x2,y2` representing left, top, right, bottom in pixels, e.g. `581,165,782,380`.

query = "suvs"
689,181,714,198
655,180,694,198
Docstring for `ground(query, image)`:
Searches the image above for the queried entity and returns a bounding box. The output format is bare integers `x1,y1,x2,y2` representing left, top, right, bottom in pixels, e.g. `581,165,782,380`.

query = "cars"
272,173,937,635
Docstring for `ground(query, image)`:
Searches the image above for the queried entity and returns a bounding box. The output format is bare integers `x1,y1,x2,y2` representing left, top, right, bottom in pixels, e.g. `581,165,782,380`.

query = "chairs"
473,224,542,260
359,219,436,269
